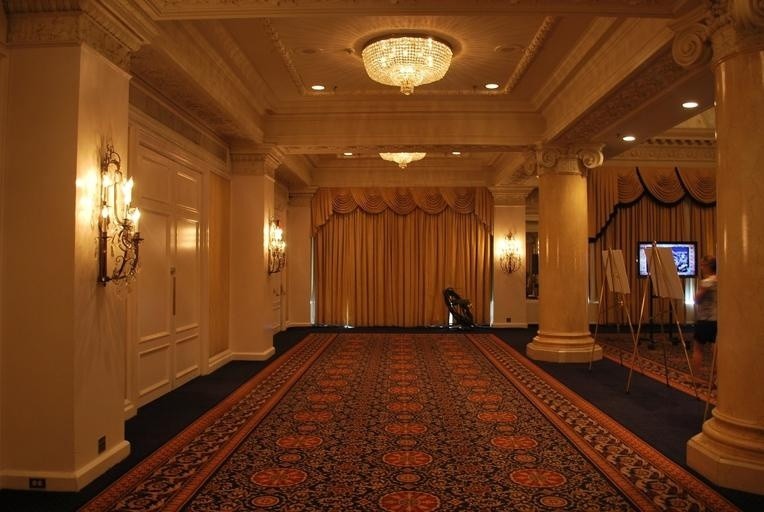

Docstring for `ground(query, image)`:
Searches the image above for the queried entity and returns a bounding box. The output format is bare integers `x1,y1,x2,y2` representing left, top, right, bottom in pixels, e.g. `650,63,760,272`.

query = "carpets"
590,331,718,408
75,332,742,512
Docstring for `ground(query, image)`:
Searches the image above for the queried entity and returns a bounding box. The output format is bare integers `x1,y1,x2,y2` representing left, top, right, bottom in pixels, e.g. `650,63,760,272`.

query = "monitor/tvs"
636,241,698,278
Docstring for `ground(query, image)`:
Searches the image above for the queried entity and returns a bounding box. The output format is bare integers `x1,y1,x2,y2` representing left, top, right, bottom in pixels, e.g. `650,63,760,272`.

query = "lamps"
360,34,453,95
269,213,286,276
93,141,145,300
378,152,427,169
500,229,523,274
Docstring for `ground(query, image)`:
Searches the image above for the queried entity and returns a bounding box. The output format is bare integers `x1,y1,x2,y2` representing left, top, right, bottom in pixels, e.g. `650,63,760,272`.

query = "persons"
691,255,717,372
